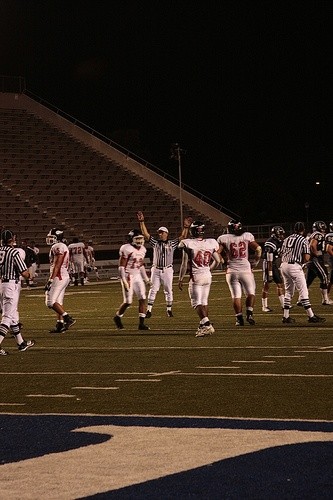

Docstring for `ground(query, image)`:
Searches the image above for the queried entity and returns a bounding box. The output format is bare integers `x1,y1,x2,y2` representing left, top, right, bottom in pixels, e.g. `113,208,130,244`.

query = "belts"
156,266,171,270
288,262,295,264
2,279,18,284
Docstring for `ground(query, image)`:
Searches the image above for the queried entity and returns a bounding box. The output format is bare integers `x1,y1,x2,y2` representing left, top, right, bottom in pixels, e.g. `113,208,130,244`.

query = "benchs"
0,108,226,249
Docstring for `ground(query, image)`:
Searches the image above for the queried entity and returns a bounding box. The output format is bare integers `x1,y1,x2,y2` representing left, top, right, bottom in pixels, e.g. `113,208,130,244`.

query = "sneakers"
261,298,333,323
48,314,77,333
0,346,7,356
195,323,216,338
17,338,35,352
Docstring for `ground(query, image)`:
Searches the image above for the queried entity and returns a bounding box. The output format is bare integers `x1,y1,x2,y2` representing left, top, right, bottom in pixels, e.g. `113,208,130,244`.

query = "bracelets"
185,227,188,229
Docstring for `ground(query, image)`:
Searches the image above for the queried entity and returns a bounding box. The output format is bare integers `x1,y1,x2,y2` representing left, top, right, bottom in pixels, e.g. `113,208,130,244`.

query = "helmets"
189,220,242,239
86,265,94,272
271,226,285,242
46,227,65,245
127,229,145,246
313,219,333,232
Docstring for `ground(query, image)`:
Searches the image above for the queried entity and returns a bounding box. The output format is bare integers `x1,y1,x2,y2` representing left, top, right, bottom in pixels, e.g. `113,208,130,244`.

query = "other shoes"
113,316,124,329
138,324,151,330
145,310,173,318
235,315,255,326
9,323,24,334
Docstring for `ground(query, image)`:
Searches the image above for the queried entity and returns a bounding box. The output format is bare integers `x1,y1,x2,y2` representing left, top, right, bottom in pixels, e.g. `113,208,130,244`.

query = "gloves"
137,211,144,222
123,280,130,292
147,279,153,286
184,217,193,228
43,278,54,292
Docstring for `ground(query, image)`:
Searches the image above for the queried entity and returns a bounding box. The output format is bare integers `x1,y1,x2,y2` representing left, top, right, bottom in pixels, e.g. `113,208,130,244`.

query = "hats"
294,222,304,231
157,226,168,233
0,230,15,240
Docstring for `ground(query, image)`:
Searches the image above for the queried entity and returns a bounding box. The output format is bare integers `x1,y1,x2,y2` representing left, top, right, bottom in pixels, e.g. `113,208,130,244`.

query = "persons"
216,219,261,327
177,221,221,337
260,221,333,322
0,229,37,356
64,238,101,287
113,229,151,331
43,227,77,334
137,210,193,319
13,240,40,291
